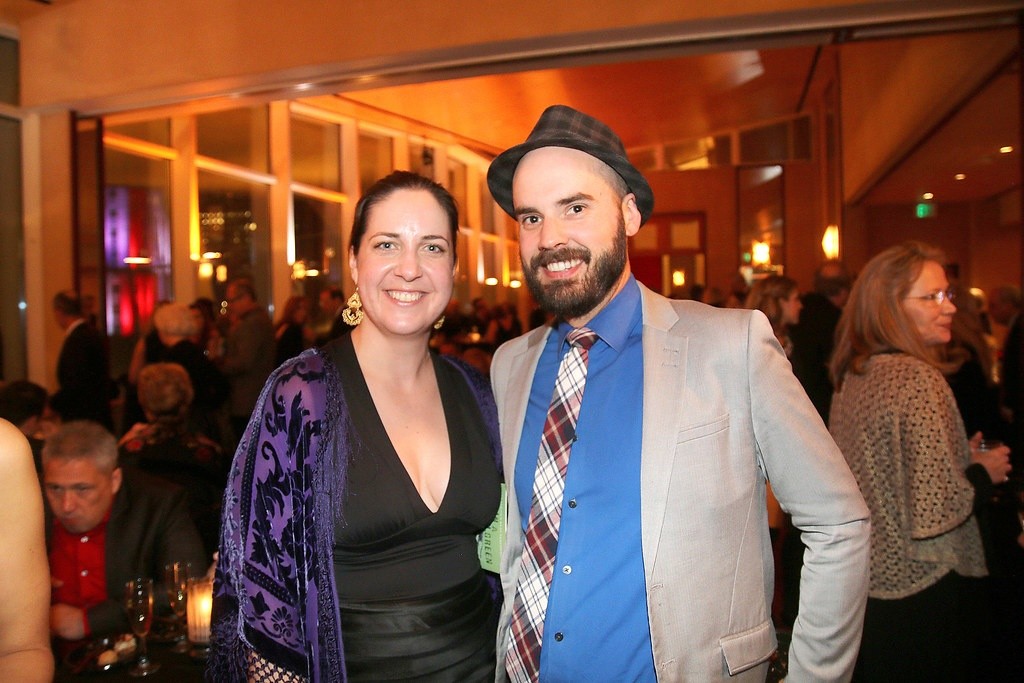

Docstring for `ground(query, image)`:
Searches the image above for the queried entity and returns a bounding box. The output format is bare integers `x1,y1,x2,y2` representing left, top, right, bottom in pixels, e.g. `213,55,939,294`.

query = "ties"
505,328,601,683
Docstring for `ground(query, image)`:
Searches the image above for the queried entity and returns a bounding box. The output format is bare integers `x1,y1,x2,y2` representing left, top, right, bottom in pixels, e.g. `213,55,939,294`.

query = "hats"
487,105,654,228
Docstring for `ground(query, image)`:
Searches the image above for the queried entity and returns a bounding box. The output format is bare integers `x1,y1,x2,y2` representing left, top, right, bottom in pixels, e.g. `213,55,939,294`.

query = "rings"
1004,476,1008,482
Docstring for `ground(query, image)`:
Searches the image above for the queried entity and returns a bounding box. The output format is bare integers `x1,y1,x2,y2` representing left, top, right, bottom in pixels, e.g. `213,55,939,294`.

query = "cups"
977,439,1010,481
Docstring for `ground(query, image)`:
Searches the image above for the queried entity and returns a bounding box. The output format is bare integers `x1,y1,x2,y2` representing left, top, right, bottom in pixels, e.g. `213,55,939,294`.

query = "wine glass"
121,577,161,676
165,559,192,653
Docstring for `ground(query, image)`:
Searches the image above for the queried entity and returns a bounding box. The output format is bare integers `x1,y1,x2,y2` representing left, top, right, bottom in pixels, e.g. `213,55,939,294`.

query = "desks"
49,636,213,683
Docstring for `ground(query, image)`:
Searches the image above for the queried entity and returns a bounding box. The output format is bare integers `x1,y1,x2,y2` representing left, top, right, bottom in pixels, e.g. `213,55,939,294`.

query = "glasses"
903,288,954,305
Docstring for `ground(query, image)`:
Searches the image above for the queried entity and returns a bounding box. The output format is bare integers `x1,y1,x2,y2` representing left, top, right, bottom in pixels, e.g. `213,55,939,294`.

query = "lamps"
186,582,213,659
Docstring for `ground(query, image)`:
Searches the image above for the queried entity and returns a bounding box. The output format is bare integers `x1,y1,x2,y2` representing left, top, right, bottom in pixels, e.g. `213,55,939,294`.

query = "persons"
0,274,549,683
207,168,503,683
487,106,871,683
668,238,1024,683
